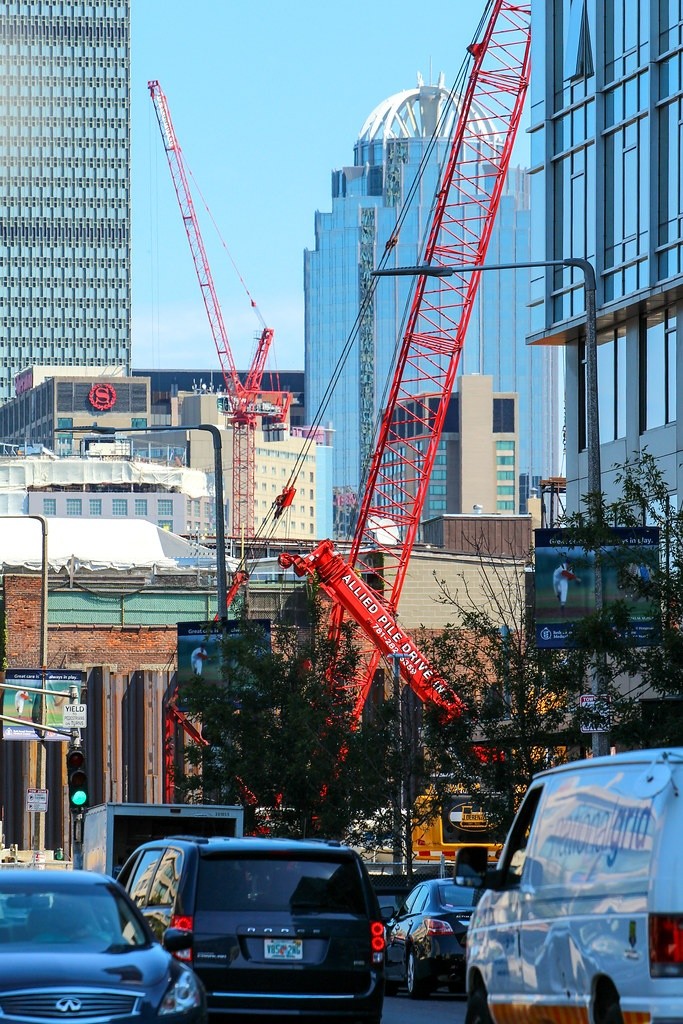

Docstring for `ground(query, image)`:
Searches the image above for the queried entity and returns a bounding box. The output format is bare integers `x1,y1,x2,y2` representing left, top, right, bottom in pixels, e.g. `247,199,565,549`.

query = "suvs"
117,838,386,1024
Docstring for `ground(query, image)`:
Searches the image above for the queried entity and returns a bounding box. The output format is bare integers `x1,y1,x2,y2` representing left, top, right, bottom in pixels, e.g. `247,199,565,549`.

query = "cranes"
162,0,534,837
147,78,275,547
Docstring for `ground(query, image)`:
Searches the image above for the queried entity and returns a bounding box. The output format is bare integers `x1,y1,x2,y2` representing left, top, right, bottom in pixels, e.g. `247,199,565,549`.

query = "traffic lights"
66,750,90,808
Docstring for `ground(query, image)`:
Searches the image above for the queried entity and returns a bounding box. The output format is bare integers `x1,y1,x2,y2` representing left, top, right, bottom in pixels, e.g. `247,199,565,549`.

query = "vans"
463,746,683,1023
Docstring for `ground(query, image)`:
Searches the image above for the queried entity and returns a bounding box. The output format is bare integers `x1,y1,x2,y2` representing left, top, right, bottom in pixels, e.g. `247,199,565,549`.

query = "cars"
382,877,488,998
0,867,210,1024
